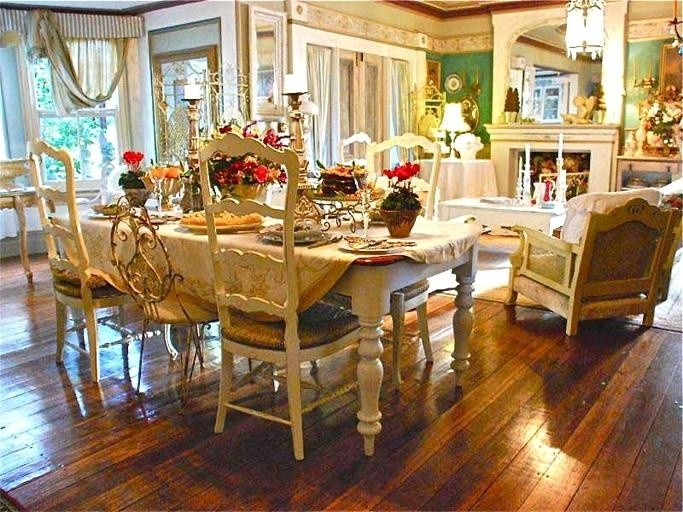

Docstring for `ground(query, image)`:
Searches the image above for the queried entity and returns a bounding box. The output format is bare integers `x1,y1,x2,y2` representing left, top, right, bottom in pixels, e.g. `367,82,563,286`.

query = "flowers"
640,104,680,148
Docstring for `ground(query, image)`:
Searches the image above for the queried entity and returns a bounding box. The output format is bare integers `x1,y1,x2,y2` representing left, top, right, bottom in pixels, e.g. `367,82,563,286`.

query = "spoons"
307,234,343,249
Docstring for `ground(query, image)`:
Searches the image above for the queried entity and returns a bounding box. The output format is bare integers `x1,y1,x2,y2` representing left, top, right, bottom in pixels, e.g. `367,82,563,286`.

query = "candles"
184,85,200,98
284,73,307,94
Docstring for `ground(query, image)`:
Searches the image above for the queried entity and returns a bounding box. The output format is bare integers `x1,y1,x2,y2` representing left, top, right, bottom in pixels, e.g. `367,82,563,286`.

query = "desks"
0,191,56,282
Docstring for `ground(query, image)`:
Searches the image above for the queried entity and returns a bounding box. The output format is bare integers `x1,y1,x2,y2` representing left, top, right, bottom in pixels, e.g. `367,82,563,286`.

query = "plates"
444,74,463,94
339,237,404,253
87,210,126,218
260,224,327,243
180,215,266,234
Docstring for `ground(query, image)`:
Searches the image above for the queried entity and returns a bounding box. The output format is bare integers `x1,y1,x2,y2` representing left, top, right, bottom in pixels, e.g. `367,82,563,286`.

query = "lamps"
565,1,605,60
461,64,481,96
633,58,655,88
667,1,683,48
439,102,465,159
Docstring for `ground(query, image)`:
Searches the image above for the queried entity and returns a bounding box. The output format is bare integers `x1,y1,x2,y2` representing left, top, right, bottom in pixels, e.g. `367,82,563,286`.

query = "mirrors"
505,17,603,124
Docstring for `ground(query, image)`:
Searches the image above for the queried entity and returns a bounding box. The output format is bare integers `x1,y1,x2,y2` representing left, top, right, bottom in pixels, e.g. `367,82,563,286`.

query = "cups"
592,108,606,124
498,112,523,124
